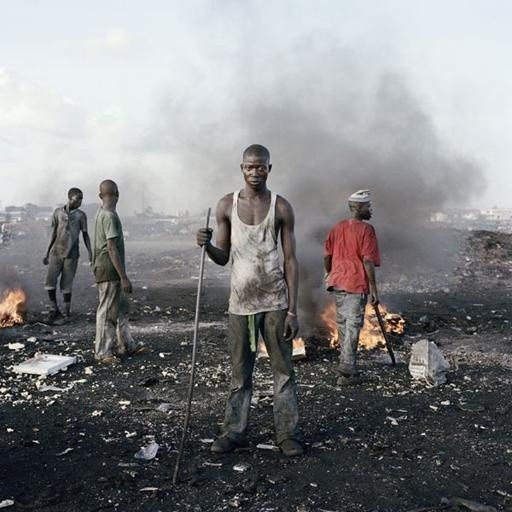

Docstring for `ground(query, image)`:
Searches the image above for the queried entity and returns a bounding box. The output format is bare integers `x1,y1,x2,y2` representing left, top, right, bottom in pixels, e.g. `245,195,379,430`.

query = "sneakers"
210,432,248,453
279,439,303,456
42,307,71,325
98,357,113,365
336,364,361,386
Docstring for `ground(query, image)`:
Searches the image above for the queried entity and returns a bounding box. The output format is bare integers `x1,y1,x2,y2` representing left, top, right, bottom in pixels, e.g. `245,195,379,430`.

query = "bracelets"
286,311,298,318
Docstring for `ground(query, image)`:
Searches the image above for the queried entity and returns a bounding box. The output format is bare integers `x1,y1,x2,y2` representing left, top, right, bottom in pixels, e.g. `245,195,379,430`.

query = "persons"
193,143,309,458
38,187,93,326
324,187,381,380
92,178,149,363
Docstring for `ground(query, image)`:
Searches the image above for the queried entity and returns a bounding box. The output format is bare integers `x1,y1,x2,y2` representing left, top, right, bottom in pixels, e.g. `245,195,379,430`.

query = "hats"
347,189,372,203
